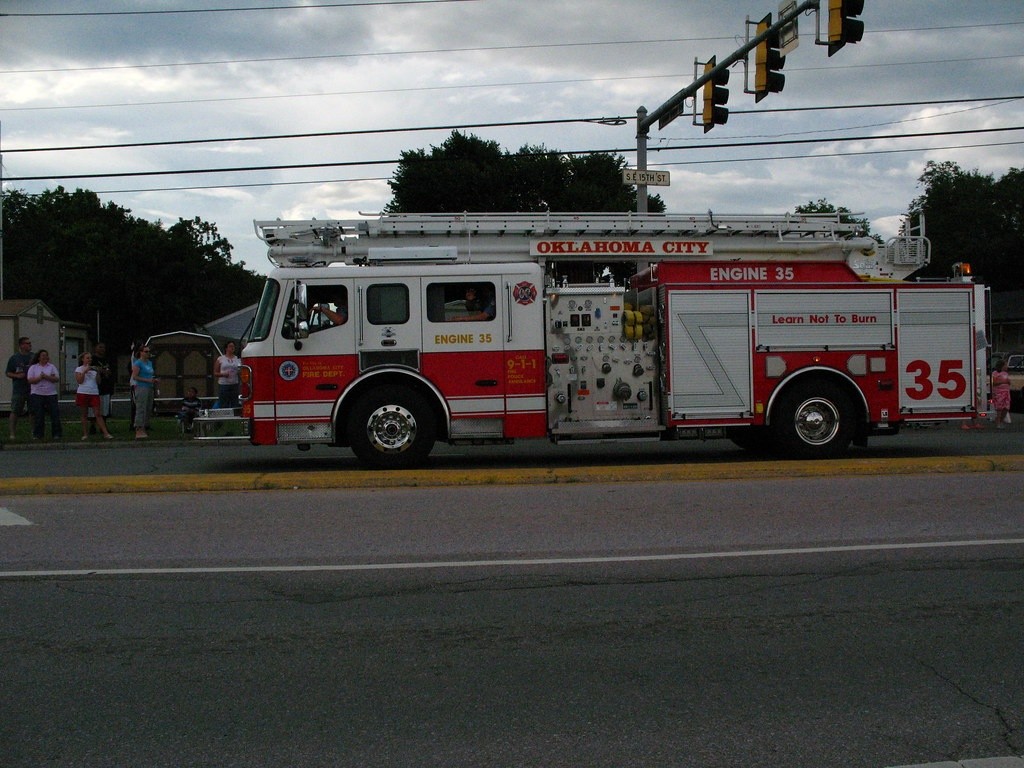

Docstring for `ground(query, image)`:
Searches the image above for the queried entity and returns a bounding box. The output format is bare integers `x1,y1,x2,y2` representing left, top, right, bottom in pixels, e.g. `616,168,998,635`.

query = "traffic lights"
755,12,785,103
827,0,865,58
703,54,729,136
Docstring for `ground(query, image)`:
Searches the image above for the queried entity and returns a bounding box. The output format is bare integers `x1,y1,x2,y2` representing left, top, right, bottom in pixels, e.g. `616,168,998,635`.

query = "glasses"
228,344,236,348
98,345,106,349
143,350,151,354
466,287,477,292
24,341,32,345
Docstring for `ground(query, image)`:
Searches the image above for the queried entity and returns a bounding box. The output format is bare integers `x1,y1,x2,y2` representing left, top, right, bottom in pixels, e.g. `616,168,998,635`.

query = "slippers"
81,433,90,441
103,434,115,440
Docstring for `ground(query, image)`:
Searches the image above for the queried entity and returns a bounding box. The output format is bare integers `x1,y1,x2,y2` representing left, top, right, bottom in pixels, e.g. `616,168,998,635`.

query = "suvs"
985,351,1024,412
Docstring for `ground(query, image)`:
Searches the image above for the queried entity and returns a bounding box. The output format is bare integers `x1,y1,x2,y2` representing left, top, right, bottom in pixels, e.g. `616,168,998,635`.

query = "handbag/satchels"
1003,410,1012,424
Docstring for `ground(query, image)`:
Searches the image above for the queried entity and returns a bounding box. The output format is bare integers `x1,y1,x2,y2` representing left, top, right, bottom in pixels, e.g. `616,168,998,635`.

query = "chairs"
175,398,202,435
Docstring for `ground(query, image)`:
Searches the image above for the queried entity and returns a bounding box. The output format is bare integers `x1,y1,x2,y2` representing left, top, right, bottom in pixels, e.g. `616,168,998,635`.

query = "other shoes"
135,429,148,439
9,434,16,441
53,435,61,442
88,427,97,435
996,422,1006,431
960,423,971,430
971,423,985,430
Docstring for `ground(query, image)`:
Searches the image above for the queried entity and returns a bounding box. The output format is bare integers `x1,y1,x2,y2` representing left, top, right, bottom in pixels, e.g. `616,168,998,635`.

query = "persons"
4,337,240,445
960,361,1011,430
311,288,495,323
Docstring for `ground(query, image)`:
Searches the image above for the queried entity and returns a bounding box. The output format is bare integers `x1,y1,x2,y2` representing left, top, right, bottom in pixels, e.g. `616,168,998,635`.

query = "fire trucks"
236,207,995,469
